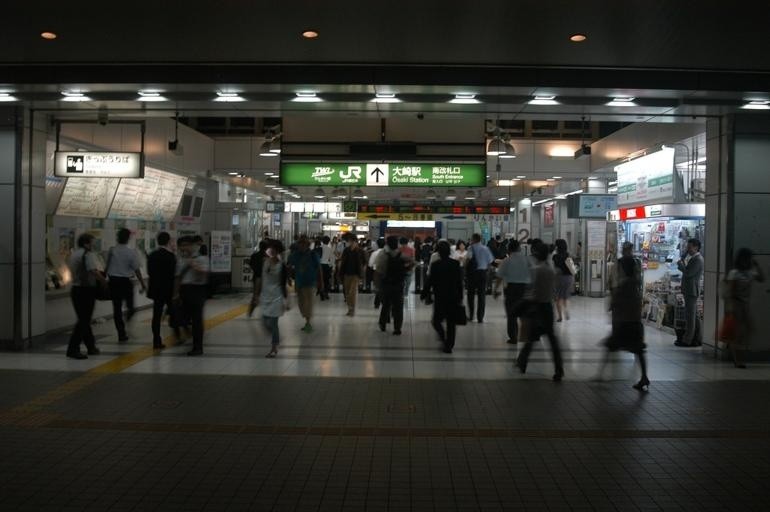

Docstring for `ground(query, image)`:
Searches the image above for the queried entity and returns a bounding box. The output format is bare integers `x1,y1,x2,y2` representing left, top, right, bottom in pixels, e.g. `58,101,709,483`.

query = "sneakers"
74,354,87,359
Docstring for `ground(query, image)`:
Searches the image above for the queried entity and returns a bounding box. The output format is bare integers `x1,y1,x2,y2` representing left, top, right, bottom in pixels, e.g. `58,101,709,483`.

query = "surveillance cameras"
575,147,591,161
168,141,184,157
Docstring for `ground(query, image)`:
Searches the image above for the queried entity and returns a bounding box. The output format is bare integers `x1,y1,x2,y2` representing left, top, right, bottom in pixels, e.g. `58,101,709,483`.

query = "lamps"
614,144,665,167
424,128,593,201
227,132,367,200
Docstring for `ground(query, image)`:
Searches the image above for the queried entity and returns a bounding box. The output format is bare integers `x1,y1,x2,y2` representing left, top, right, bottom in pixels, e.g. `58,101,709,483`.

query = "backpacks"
386,251,405,284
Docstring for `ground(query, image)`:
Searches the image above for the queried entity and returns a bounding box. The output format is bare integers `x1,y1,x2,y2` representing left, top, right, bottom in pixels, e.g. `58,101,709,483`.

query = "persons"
606,242,648,348
255,239,291,358
719,247,764,369
608,256,651,389
420,240,463,353
512,242,565,382
249,240,268,316
673,239,704,346
287,230,439,335
451,232,542,345
65,227,210,360
553,239,574,322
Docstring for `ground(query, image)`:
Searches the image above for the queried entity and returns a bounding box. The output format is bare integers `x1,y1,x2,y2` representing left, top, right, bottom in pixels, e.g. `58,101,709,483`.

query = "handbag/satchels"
565,257,577,275
465,257,478,291
165,298,188,328
96,280,111,300
456,305,467,325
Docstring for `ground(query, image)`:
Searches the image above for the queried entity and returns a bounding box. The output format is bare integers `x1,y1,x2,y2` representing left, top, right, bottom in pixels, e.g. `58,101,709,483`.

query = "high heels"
633,378,650,389
